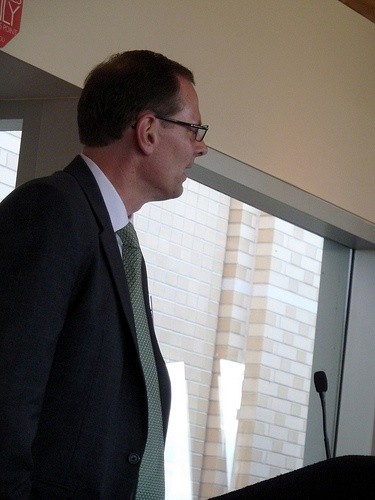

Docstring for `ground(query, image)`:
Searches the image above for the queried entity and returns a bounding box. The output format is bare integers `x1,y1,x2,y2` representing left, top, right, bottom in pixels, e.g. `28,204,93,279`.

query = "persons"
0,50,209,500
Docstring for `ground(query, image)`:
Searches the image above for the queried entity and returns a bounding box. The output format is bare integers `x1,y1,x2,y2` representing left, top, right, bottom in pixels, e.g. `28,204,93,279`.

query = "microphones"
313,371,333,459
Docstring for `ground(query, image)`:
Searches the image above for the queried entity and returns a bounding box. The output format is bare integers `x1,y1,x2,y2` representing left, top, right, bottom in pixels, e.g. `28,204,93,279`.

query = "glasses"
131,113,210,144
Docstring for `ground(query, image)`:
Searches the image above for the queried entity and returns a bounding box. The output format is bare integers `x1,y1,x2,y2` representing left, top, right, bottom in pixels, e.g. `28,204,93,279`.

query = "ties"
116,218,169,500
